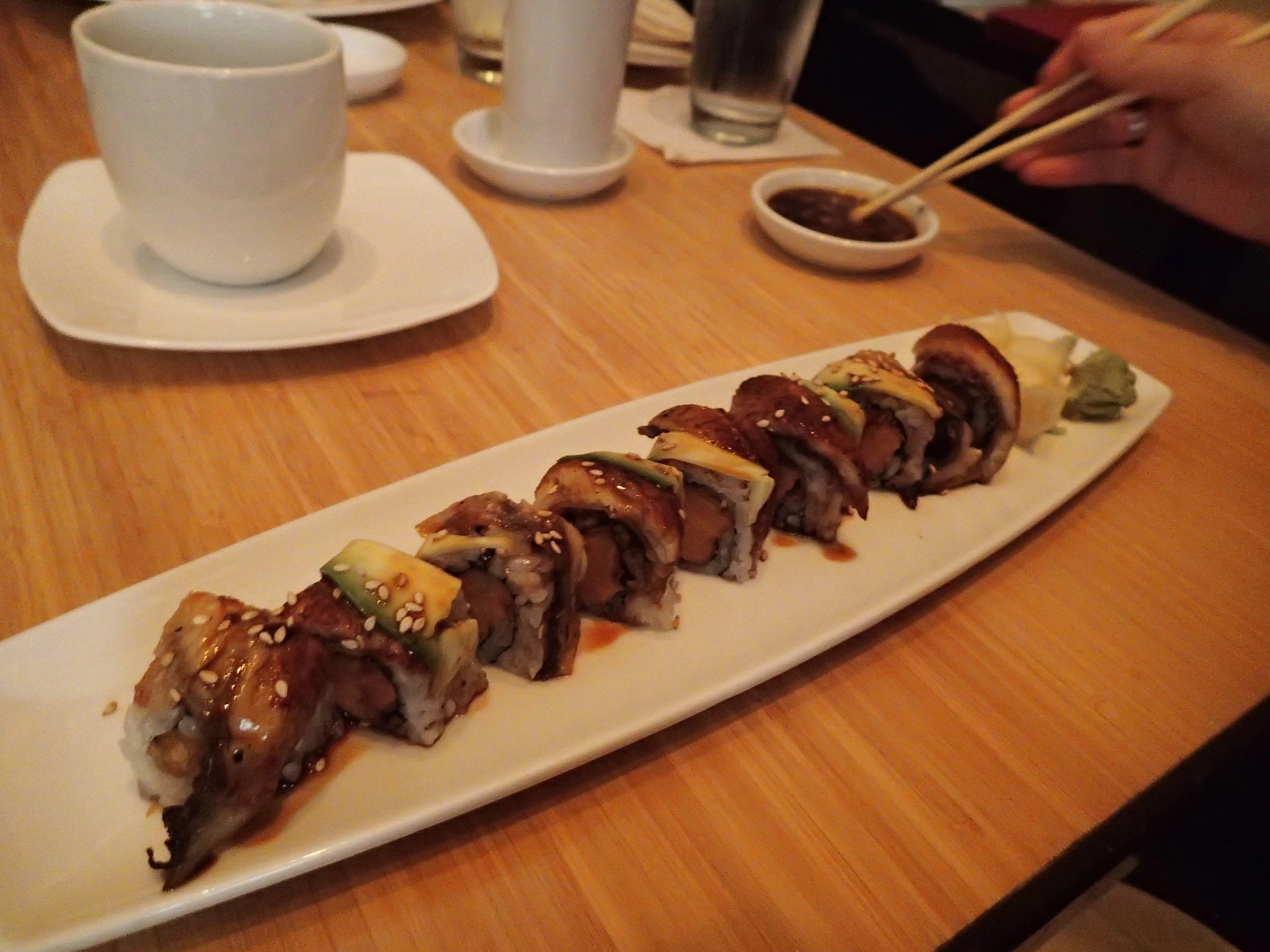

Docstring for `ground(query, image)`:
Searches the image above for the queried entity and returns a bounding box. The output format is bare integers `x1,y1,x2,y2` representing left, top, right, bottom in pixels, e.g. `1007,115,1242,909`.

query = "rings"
1123,105,1147,147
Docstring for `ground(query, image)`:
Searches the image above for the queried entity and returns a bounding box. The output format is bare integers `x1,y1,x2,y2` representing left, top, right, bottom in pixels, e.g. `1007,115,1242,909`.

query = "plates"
3,308,1187,952
324,23,407,104
16,150,498,351
751,164,942,269
454,105,637,200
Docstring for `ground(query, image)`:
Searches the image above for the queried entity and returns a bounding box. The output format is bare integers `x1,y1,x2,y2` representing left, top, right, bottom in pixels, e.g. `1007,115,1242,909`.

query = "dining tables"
3,0,1269,951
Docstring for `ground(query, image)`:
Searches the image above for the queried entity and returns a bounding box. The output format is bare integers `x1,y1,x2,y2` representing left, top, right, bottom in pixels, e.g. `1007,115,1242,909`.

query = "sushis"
115,309,1083,892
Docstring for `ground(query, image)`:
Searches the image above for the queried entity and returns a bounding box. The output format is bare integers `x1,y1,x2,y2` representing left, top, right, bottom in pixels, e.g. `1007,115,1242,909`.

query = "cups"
685,0,821,147
71,0,350,289
505,1,637,168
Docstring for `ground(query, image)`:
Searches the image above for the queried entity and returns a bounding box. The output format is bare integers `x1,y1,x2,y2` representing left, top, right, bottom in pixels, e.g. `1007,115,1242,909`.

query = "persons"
996,0,1270,243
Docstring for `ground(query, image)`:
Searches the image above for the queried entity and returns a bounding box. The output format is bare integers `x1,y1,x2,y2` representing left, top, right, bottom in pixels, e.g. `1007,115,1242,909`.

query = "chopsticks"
840,0,1269,210
